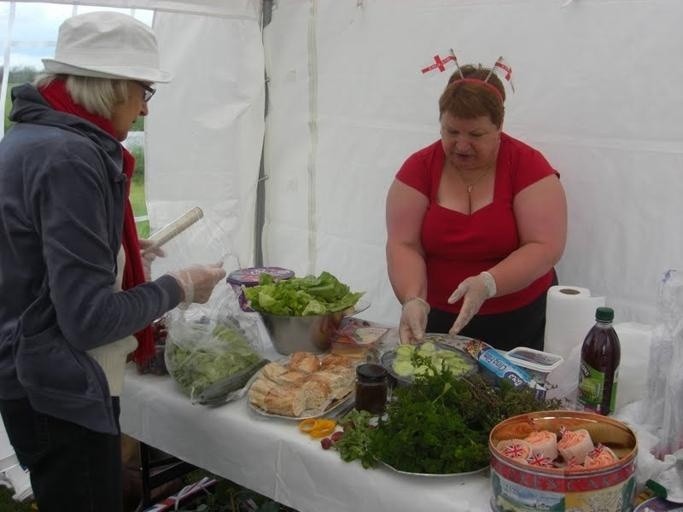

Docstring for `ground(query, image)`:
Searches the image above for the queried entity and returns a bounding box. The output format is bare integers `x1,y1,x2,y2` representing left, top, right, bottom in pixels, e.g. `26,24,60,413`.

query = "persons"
385,62,567,351
0,12,227,512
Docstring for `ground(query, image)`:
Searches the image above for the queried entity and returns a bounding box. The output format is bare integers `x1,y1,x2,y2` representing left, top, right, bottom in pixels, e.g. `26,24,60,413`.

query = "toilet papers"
543,284,607,362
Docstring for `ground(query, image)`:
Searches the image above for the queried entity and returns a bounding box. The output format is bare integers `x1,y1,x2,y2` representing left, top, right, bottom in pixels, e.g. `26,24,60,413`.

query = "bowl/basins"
254,300,345,355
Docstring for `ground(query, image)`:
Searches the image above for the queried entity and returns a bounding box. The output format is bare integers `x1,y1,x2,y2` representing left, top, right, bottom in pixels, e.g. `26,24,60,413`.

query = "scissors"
299,402,356,437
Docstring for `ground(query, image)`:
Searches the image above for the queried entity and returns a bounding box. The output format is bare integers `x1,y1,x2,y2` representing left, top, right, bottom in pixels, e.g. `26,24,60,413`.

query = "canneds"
354,364,387,417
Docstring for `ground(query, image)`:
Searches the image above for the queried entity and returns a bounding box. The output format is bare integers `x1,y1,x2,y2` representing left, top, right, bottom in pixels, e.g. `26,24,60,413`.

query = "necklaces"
458,170,489,193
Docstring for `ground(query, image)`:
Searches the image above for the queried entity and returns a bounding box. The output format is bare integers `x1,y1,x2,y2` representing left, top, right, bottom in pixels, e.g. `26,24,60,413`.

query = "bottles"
352,362,391,417
575,304,623,417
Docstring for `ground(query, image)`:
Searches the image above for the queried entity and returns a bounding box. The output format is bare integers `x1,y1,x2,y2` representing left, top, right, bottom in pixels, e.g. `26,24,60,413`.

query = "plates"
246,349,367,425
378,331,498,388
369,445,492,482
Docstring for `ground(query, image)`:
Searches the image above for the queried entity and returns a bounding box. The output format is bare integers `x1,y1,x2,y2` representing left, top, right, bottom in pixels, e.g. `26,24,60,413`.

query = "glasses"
129,79,157,102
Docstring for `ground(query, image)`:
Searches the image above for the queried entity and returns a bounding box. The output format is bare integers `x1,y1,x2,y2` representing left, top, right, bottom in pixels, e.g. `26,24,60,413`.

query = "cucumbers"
199,357,270,405
393,342,469,378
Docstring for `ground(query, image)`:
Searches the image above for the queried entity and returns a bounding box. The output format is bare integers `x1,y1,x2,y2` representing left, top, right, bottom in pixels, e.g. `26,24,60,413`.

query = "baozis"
496,425,619,470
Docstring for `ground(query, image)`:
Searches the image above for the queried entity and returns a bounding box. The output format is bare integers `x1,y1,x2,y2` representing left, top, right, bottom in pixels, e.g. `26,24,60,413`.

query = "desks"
87,311,492,509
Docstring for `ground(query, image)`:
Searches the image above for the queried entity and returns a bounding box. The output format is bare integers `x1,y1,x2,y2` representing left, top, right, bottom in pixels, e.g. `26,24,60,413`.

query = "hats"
41,12,174,83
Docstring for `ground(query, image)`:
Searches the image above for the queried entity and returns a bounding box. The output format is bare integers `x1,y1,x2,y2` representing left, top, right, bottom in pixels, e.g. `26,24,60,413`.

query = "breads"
247,351,367,418
